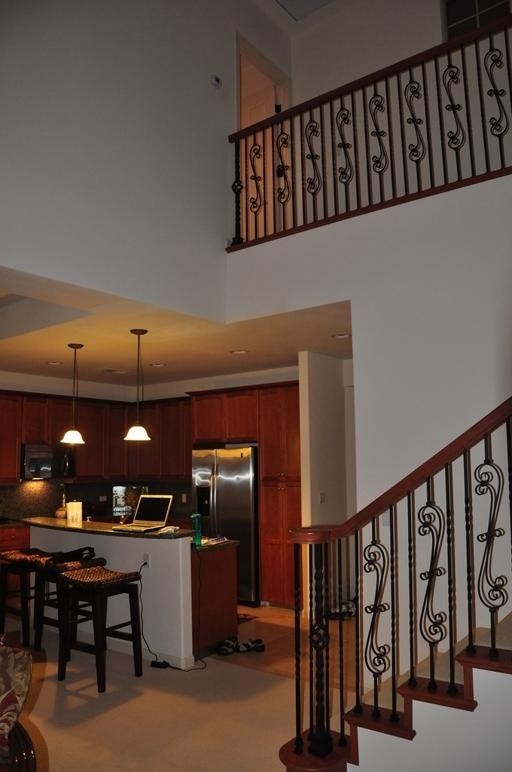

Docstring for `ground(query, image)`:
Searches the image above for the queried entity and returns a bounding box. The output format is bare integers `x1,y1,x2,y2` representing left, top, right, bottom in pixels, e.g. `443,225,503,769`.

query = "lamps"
61,343,87,445
123,328,152,442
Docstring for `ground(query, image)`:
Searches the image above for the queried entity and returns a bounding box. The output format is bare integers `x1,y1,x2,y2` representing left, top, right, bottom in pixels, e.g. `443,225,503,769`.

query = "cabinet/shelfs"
18,393,75,479
126,402,161,479
1,525,30,626
261,380,300,482
158,397,193,478
23,517,238,663
185,386,261,441
1,391,19,485
75,397,124,480
259,480,303,610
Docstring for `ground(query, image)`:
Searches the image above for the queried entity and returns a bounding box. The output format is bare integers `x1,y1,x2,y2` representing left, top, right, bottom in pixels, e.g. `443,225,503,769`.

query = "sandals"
237,638,264,652
217,637,239,656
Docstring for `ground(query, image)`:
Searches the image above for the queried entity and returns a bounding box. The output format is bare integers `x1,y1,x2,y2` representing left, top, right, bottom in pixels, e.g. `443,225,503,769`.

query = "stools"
1,548,48,646
35,556,105,662
57,567,144,692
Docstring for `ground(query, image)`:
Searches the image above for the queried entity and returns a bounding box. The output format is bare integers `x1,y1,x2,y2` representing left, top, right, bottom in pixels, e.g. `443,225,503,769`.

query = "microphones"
151,661,169,668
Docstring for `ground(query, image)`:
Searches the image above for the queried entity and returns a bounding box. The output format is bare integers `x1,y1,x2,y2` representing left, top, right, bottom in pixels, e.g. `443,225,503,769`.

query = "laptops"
112,495,173,533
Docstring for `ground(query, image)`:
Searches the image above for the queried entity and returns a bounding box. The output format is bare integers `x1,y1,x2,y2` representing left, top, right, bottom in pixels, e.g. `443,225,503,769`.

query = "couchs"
0,646,37,771
